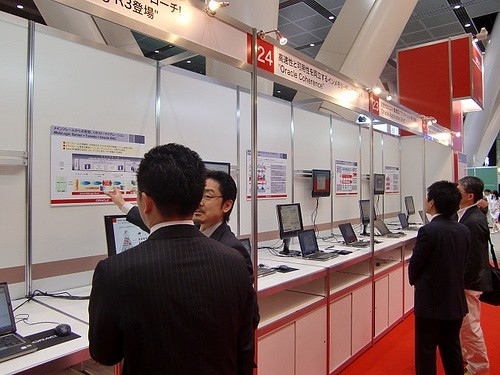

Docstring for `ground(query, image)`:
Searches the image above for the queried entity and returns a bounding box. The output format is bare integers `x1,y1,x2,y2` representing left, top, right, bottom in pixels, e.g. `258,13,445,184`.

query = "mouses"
279,265,289,271
54,324,71,336
338,250,347,254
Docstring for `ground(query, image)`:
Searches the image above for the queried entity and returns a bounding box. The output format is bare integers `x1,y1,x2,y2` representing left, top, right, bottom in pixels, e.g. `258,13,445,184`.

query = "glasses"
204,194,222,199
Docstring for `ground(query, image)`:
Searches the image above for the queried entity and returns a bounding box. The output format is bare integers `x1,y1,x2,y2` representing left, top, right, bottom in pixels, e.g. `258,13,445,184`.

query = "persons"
87,142,260,375
103,171,254,285
408,180,471,375
458,176,491,375
476,189,500,233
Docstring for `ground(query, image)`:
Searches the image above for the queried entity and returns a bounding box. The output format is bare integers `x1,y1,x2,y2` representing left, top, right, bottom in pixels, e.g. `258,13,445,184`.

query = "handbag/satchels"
479,264,500,306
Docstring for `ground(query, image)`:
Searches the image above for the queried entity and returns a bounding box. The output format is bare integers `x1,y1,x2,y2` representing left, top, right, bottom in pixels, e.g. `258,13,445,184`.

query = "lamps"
385,91,392,100
206,0,229,17
263,30,287,45
427,118,437,125
452,131,460,137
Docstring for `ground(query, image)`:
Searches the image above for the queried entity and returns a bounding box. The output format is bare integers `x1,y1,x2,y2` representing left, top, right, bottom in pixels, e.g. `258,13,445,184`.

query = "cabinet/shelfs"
0,299,122,375
33,286,92,324
255,221,421,375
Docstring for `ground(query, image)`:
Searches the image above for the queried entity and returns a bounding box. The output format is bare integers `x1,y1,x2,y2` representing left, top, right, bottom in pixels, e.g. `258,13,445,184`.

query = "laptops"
0,281,38,363
238,238,276,278
297,229,338,261
374,218,404,238
398,213,419,231
338,223,370,248
419,211,429,224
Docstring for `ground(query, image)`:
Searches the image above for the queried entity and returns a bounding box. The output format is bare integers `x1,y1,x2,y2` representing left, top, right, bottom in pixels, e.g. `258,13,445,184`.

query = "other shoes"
491,230,498,234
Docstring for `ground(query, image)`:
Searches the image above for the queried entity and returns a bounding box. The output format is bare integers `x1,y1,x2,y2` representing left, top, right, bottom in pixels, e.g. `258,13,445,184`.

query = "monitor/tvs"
405,196,415,224
312,169,331,197
276,203,303,255
358,200,377,236
374,174,385,194
104,215,150,257
